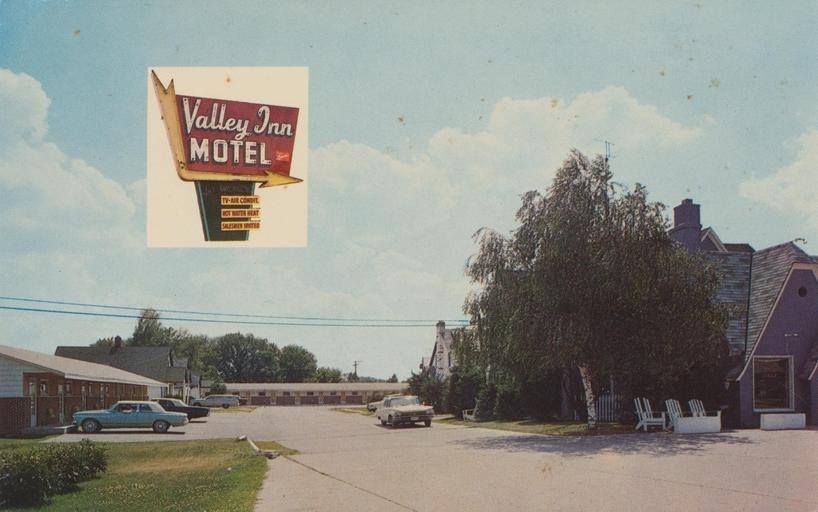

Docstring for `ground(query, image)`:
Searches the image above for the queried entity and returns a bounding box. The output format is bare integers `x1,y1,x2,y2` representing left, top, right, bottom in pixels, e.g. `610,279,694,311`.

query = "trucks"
190,394,239,410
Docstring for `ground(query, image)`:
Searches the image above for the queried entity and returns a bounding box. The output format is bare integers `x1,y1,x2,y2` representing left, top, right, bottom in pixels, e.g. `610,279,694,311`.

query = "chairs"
633,397,668,433
688,397,708,427
664,398,688,431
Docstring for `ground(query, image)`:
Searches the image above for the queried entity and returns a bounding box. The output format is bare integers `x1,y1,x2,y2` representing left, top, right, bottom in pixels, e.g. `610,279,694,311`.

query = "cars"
375,395,435,427
72,400,188,433
236,395,247,405
367,394,403,413
149,396,210,420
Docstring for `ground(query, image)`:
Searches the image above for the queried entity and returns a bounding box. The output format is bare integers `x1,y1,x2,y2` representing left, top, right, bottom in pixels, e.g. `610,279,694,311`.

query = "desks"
673,416,722,433
759,412,806,430
